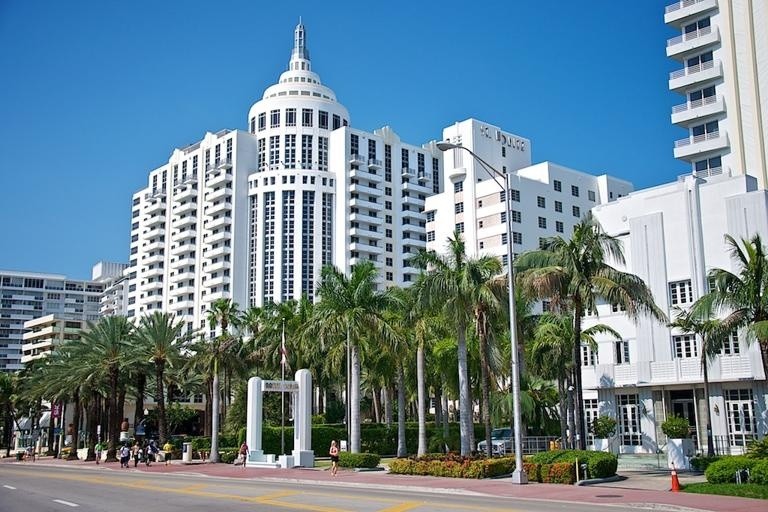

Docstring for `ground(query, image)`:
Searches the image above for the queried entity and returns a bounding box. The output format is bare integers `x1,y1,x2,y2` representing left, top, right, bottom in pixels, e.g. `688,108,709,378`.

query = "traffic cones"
669,460,685,493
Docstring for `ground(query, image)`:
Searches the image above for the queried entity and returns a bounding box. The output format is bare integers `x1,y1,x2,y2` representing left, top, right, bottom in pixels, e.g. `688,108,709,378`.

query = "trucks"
472,426,541,459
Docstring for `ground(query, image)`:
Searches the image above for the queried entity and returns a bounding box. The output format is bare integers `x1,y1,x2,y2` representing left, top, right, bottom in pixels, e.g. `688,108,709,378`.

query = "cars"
171,434,186,439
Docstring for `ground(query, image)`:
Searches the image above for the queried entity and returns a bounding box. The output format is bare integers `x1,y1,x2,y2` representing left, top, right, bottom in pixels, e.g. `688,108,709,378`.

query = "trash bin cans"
183,442,192,461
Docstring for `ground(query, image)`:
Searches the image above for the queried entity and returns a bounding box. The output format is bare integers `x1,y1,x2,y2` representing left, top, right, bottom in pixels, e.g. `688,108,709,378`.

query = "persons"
145,441,158,466
163,439,173,466
26,444,33,458
131,440,141,467
329,439,339,477
94,439,103,464
240,440,250,471
119,443,131,468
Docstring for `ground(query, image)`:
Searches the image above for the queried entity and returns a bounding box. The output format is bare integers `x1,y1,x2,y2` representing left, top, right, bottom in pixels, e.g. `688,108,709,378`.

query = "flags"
279,330,292,369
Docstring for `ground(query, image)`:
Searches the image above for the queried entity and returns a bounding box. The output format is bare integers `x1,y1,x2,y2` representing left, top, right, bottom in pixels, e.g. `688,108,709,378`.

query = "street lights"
433,138,529,489
